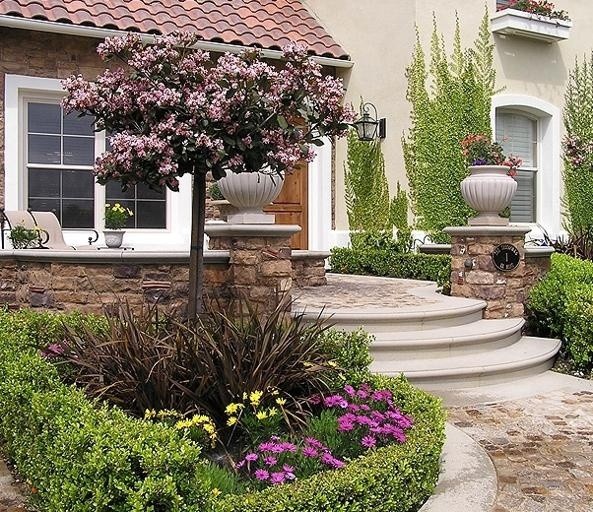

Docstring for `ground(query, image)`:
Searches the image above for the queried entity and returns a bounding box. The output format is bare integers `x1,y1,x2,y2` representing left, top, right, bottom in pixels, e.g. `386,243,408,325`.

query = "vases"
486,8,574,46
457,164,517,224
102,229,126,249
220,160,285,224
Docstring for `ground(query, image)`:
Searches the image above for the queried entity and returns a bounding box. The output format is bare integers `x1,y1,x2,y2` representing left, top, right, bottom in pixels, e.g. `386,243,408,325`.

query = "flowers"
496,0,574,24
457,127,526,175
102,201,134,228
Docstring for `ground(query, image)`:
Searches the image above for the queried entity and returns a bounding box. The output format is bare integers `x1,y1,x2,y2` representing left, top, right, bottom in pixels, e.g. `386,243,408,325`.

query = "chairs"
1,208,49,249
27,208,99,251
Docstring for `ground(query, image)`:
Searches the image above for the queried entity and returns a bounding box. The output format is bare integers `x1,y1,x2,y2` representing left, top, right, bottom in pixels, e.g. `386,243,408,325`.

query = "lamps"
351,102,388,142
